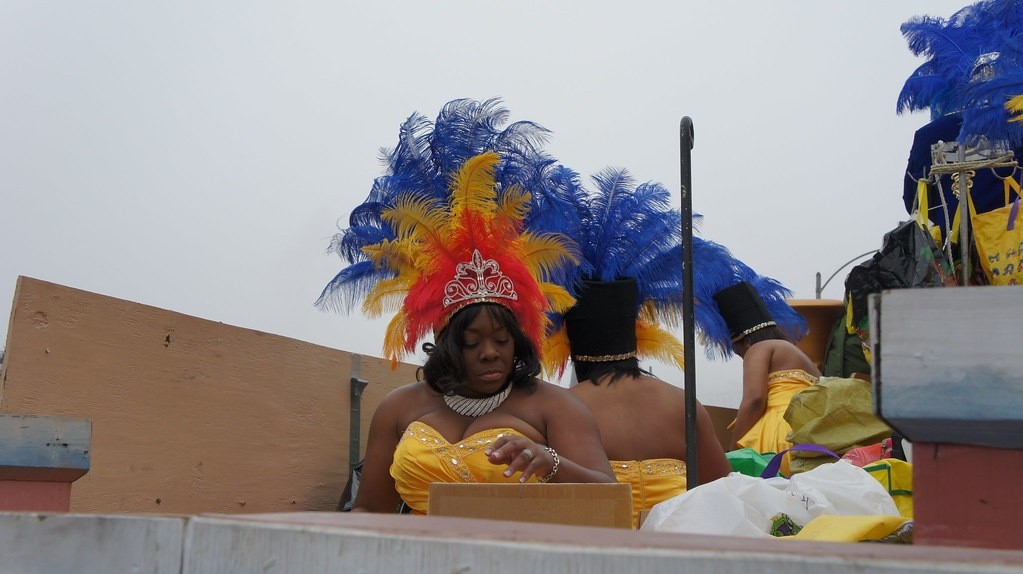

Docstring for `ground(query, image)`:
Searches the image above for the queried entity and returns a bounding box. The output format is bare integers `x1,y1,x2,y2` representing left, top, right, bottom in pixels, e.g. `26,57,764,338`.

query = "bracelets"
535,447,559,482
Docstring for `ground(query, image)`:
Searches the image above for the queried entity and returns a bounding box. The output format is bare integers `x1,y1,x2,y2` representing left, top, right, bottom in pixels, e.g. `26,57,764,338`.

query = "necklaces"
438,380,513,418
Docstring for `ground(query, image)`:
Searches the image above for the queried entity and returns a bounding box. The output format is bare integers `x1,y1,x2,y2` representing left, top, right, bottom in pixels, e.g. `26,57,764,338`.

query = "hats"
534,166,703,361
681,233,777,342
311,96,596,342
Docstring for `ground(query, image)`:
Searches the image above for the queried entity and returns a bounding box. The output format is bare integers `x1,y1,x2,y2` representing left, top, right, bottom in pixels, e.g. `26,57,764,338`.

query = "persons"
564,275,733,529
713,281,823,480
350,248,619,516
903,52,1023,261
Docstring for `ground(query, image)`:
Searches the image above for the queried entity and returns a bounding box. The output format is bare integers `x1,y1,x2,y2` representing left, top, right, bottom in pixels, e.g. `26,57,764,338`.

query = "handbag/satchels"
968,175,1023,285
845,180,943,369
942,200,989,286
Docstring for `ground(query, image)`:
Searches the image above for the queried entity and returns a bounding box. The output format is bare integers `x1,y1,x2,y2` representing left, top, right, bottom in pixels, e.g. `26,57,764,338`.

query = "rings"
522,448,532,461
